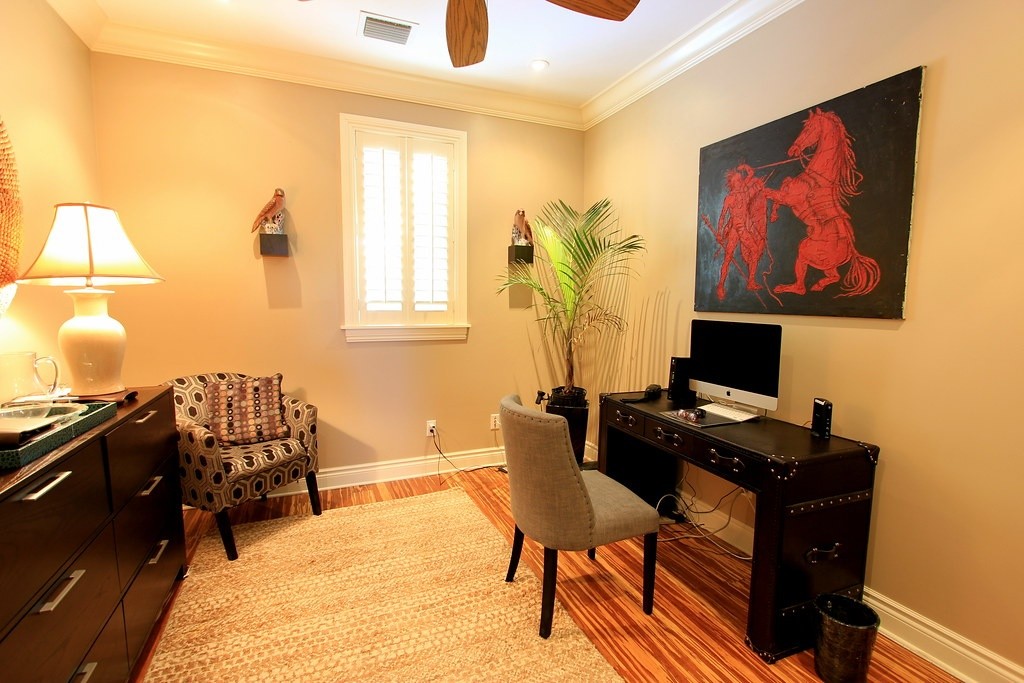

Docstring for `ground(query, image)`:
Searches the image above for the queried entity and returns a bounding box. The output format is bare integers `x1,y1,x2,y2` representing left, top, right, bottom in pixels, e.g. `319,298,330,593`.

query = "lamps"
16,203,164,396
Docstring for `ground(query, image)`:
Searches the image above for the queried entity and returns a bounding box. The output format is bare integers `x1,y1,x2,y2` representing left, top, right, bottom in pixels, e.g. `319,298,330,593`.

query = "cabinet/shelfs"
1,386,186,683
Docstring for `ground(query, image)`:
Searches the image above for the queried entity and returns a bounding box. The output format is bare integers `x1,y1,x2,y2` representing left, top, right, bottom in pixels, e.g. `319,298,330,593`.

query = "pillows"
203,373,288,447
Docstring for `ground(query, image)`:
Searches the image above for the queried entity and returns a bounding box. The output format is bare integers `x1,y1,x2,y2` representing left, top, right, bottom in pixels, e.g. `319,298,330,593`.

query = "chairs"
499,394,659,638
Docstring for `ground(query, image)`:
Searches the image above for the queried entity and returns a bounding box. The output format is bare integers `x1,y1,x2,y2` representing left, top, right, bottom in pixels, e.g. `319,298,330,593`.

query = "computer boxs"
666,357,696,401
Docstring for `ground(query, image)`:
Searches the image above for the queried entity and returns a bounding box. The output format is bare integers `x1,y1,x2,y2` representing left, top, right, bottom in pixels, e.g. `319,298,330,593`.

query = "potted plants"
495,199,647,464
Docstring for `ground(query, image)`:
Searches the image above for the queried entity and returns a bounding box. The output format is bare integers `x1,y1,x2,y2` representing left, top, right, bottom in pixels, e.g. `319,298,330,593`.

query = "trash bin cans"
814,593,880,683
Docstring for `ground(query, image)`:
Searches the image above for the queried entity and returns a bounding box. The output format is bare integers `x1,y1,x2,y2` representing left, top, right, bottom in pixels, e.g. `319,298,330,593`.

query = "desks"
598,389,879,659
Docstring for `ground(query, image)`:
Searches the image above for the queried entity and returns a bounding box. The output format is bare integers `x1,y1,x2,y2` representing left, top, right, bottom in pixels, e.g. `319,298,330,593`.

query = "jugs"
1,350,60,438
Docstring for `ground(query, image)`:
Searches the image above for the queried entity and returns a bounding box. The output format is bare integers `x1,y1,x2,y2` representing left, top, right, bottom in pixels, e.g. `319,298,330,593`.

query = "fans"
447,0,640,68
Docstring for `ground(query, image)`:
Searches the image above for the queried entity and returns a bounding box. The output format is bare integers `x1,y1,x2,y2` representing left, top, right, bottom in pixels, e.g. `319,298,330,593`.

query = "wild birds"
251,188,285,233
514,209,534,247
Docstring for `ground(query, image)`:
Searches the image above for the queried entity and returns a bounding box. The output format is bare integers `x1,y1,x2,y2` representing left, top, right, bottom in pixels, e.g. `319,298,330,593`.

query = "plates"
1,402,89,431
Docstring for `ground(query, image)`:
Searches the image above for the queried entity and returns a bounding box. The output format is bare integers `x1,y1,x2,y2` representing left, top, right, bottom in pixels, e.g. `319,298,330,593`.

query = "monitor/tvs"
688,318,783,412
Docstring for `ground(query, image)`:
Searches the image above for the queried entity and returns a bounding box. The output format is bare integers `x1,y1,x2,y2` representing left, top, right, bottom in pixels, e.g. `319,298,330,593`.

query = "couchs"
159,373,322,561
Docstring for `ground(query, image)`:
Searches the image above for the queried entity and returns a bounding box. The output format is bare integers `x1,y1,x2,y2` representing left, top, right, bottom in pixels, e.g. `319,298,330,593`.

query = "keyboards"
696,402,760,423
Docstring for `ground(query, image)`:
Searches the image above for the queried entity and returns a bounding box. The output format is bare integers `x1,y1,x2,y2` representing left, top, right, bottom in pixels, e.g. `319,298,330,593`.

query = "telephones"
644,384,661,398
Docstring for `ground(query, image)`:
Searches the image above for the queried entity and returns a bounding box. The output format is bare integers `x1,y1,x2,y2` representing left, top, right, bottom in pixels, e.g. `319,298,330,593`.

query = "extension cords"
658,509,686,524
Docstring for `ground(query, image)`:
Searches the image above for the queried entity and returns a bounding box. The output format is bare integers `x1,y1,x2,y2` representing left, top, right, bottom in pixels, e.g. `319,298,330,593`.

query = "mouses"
693,408,706,419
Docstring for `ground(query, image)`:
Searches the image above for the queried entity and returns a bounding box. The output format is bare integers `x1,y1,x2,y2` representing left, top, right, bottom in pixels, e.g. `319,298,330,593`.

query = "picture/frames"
694,66,925,319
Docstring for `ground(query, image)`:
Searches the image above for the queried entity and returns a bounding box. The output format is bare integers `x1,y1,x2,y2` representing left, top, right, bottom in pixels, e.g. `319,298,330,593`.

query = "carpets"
143,488,629,683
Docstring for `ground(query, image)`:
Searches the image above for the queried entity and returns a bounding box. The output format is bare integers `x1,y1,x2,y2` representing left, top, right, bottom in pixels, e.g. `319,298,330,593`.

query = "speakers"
810,398,832,440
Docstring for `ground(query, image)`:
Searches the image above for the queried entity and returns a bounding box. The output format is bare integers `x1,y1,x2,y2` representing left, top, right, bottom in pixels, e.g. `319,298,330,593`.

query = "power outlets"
739,486,753,499
491,414,499,430
425,420,437,437
